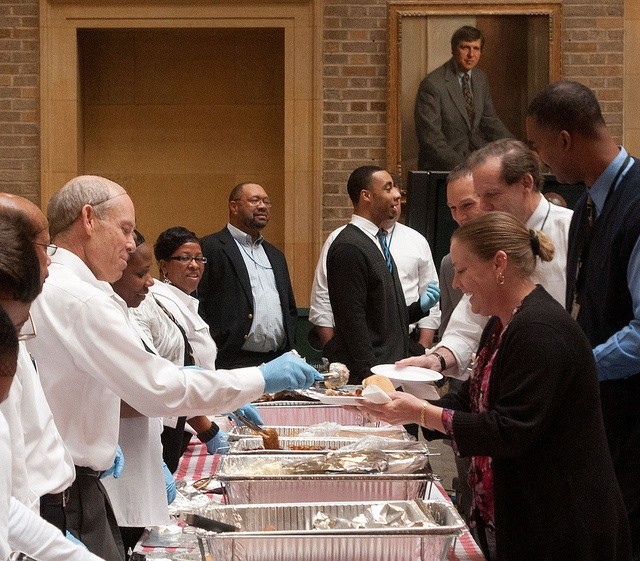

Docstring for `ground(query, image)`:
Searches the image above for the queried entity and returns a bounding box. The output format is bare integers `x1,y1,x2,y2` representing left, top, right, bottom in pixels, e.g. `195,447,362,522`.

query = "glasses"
16,310,37,342
22,241,58,255
232,196,273,208
160,255,207,265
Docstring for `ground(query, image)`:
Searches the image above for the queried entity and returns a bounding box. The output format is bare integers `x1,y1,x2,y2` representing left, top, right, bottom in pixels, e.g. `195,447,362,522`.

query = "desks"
125,384,484,557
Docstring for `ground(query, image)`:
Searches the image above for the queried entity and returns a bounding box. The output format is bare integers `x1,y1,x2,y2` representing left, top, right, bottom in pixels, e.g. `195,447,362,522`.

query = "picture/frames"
386,0,565,204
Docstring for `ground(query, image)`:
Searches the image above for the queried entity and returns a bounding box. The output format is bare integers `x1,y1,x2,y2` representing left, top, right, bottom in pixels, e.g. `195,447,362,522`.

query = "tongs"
230,411,271,440
317,372,341,382
185,515,241,532
183,486,229,498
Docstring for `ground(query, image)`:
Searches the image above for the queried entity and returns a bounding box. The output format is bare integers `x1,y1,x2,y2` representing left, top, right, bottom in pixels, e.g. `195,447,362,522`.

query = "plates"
370,364,444,382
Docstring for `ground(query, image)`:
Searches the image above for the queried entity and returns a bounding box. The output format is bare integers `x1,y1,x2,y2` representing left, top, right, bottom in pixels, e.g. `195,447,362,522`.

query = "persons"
193,182,298,378
326,167,441,384
413,26,543,177
393,136,576,381
0,301,20,405
23,174,326,560
437,159,482,342
308,180,441,389
525,80,639,385
150,225,263,465
344,209,623,561
1,190,78,561
116,225,177,544
0,209,38,345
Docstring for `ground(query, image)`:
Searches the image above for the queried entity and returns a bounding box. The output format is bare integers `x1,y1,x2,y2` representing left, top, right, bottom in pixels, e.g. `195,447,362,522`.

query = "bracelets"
418,400,429,431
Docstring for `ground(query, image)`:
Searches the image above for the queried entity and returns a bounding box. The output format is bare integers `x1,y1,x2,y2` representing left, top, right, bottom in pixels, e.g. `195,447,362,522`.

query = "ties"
378,230,392,274
570,193,597,327
461,71,475,126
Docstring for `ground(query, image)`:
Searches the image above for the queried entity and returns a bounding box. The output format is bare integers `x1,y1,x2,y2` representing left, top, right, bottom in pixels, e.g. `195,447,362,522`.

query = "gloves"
420,279,441,313
205,431,230,455
258,351,326,394
163,459,176,504
236,403,264,427
102,444,125,480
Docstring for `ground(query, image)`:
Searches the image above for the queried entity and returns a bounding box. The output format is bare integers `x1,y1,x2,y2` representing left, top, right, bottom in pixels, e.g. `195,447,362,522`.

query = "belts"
44,488,71,508
72,465,104,479
